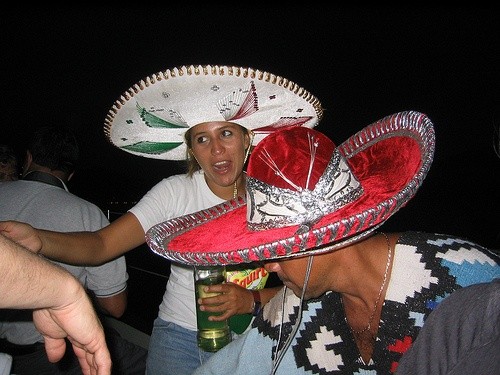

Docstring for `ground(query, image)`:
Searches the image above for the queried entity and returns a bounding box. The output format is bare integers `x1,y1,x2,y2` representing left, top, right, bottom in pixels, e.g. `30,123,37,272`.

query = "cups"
194,265,231,352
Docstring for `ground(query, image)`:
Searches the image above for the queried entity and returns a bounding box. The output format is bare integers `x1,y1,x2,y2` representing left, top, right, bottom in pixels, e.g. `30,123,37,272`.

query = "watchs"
251,289,262,317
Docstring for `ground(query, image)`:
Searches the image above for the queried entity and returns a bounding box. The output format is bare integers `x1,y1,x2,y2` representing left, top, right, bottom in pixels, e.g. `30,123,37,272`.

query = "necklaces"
340,231,392,335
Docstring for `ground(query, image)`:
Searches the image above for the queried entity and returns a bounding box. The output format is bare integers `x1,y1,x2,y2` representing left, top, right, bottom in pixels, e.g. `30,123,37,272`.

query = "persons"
193,126,500,375
394,278,500,375
1,233,112,375
0,90,269,374
1,102,128,374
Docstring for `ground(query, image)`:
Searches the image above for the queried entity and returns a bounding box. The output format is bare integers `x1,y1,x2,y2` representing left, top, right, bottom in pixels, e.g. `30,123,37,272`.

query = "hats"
103,65,323,161
145,111,434,266
